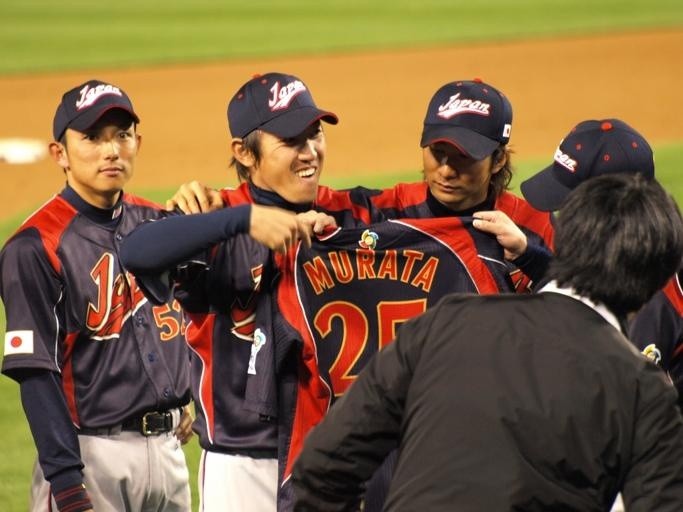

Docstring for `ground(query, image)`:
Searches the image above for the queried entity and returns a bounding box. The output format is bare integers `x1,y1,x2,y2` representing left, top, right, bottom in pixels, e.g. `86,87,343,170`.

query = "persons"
119,74,383,511
472,117,681,411
285,172,682,510
0,78,198,512
164,81,553,299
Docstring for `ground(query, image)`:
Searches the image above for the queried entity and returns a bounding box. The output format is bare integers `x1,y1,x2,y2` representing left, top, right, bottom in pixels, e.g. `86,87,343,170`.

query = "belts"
122,409,185,436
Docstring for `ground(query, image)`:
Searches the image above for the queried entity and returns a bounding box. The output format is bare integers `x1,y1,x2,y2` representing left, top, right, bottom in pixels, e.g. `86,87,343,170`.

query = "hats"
521,119,655,211
53,80,140,141
420,80,512,161
228,73,338,139
